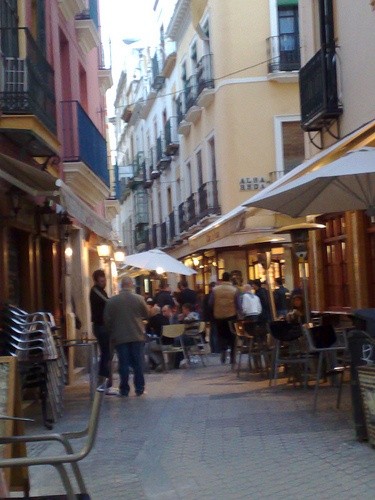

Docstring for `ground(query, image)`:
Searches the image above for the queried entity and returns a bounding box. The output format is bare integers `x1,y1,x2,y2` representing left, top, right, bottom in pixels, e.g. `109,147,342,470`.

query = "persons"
90,270,307,395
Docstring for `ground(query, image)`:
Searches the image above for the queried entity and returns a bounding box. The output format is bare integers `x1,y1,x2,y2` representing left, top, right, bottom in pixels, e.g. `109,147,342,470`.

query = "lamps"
113,246,129,264
96,239,113,263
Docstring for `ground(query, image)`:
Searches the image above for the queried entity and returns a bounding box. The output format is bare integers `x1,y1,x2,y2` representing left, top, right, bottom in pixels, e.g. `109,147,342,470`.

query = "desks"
311,310,353,386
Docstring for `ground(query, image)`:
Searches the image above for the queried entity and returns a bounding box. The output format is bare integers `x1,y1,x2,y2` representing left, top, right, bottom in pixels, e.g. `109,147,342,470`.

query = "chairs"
0,303,348,500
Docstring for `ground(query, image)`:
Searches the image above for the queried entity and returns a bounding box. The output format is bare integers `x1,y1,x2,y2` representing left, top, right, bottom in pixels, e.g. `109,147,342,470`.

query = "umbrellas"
242,147,375,218
117,249,198,279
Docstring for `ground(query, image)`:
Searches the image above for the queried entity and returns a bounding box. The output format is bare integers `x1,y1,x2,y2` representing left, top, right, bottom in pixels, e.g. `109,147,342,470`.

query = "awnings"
188,119,375,248
